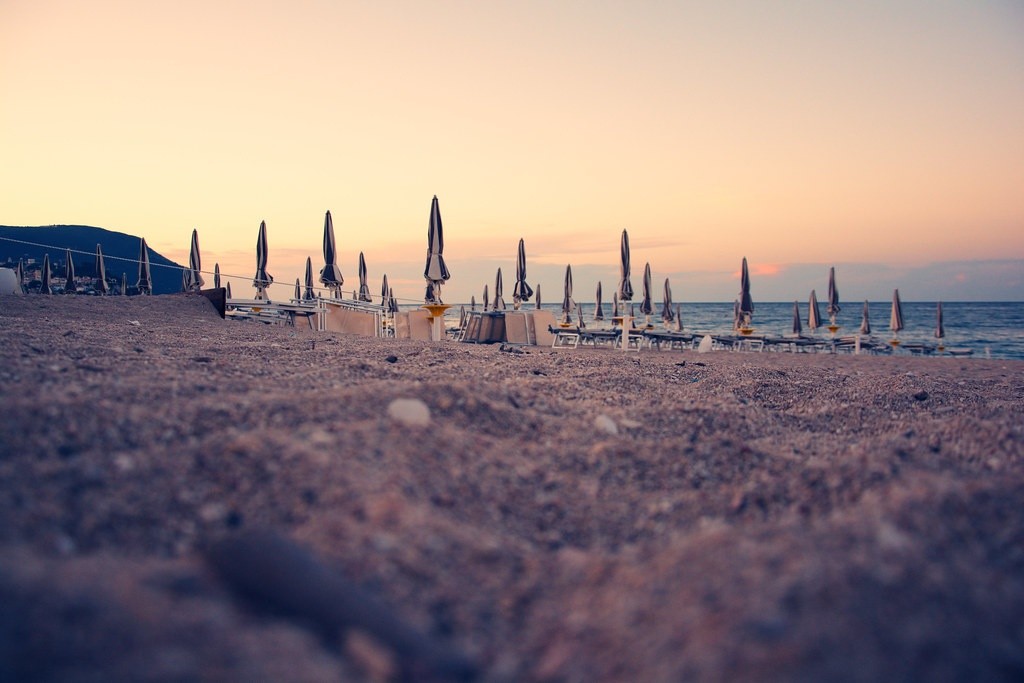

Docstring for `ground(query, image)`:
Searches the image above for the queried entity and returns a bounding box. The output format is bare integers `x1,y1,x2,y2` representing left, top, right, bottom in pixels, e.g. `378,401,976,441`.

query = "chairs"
199,287,974,356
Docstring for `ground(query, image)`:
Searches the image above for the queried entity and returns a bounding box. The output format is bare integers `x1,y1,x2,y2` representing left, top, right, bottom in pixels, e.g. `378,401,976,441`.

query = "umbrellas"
828,267,840,325
294,257,315,304
593,281,603,322
860,300,870,334
380,274,398,313
352,252,372,303
807,290,822,334
562,265,577,324
182,221,274,300
460,239,540,328
423,196,450,307
611,228,633,325
639,262,656,326
38,254,52,294
733,257,754,331
889,288,903,339
319,210,343,299
935,303,945,346
93,243,109,295
15,257,27,294
661,279,682,331
578,303,586,328
792,301,804,336
121,237,151,295
63,249,76,294
